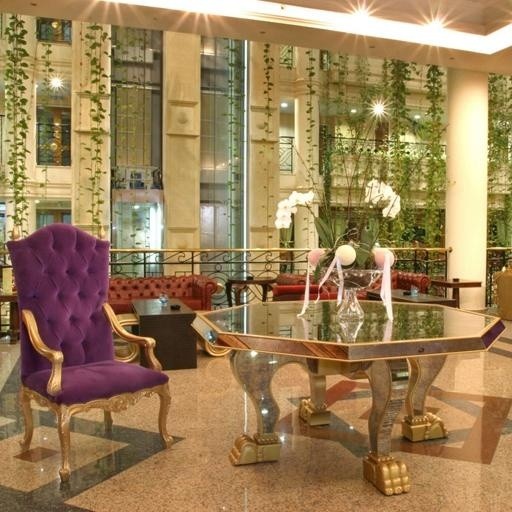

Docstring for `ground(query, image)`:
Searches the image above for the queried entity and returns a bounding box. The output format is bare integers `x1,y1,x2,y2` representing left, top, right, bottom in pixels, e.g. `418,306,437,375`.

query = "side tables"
432,274,481,308
224,272,273,308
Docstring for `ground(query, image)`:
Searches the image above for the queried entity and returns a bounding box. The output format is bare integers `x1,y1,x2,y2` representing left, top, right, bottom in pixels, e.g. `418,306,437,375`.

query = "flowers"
268,162,406,273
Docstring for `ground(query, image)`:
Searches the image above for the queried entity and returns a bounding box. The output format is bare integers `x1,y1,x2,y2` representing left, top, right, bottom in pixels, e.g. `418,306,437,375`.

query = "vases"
322,254,385,319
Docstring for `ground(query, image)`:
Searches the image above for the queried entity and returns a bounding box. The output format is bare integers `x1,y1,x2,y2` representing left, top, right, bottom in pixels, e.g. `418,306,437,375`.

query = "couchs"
84,275,218,320
274,270,435,301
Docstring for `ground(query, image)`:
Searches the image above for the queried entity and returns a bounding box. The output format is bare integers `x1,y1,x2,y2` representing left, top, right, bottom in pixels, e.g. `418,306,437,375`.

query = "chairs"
3,221,177,485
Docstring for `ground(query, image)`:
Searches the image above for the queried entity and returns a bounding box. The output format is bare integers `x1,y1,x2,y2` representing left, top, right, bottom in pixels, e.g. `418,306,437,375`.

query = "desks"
190,296,507,499
